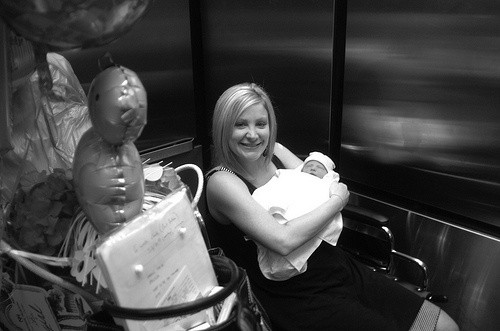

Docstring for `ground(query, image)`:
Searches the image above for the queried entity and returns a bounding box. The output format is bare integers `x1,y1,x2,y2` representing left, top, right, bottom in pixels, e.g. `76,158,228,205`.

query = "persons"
244,152,343,281
200,82,459,331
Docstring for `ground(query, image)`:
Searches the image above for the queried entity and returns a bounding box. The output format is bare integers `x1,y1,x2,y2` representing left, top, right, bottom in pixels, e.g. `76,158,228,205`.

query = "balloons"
70,66,148,236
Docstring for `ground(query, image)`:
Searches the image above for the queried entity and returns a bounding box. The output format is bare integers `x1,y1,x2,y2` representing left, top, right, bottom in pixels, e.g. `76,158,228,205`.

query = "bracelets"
329,194,343,212
